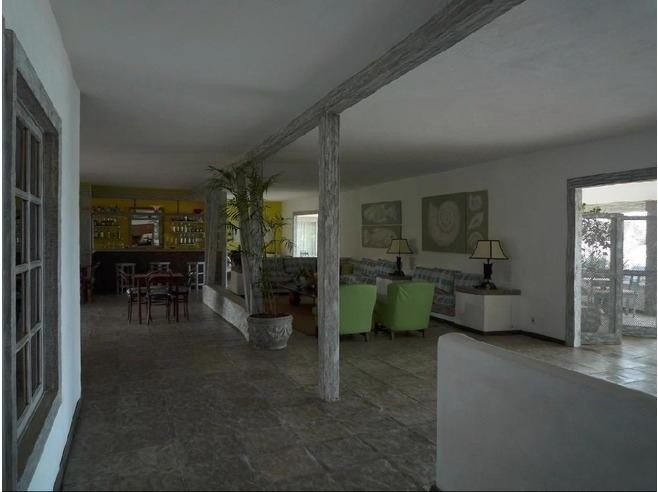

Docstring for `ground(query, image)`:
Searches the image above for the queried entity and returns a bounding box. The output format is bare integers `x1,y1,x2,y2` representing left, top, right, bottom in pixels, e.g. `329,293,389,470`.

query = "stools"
116,261,206,300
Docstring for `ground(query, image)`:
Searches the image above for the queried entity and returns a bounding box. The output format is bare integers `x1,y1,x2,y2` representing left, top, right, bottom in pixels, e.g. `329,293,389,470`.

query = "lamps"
385,237,414,277
468,239,509,291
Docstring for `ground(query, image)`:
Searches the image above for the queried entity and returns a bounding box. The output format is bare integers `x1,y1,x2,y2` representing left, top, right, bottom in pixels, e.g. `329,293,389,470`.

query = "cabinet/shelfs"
455,290,518,336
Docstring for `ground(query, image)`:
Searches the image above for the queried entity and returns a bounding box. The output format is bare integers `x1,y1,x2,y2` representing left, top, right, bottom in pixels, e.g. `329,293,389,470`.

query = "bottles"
94,216,121,239
170,221,203,245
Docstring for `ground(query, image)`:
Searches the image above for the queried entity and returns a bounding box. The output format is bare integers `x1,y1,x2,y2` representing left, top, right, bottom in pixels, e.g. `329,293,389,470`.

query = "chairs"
339,281,436,342
113,266,191,326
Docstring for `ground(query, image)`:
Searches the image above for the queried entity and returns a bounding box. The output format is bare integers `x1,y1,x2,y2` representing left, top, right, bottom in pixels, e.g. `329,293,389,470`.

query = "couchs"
339,257,483,317
264,257,317,294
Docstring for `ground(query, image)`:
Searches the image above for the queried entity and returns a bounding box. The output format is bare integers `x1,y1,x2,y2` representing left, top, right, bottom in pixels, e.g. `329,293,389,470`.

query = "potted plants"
202,160,299,351
226,249,247,295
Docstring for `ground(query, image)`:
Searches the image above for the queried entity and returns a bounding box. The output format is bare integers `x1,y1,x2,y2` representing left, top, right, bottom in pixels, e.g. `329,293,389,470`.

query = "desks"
594,289,640,319
